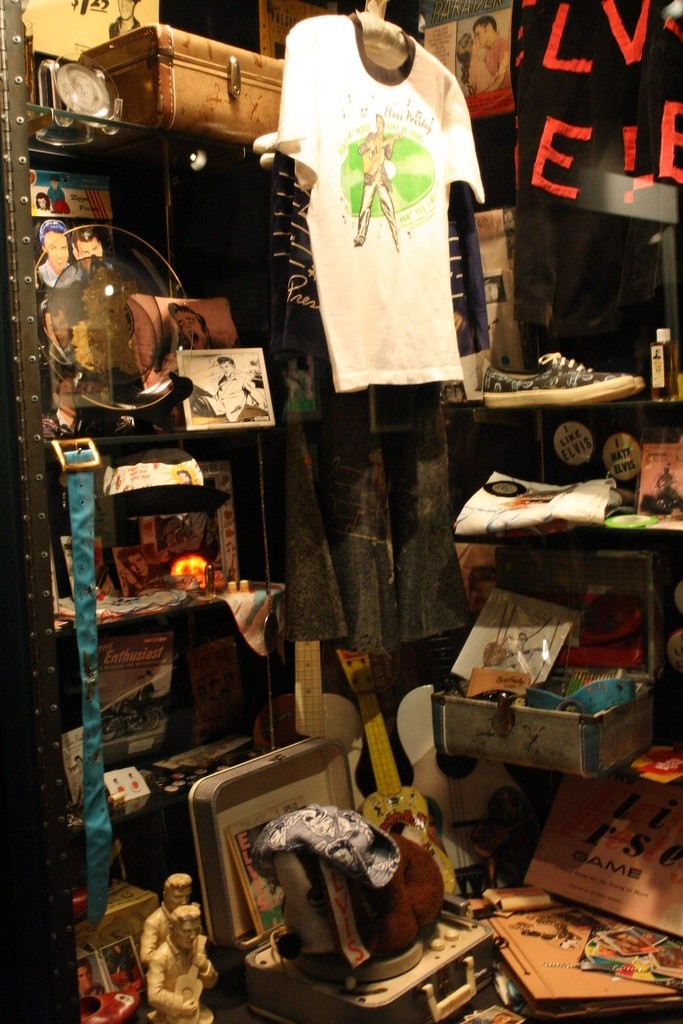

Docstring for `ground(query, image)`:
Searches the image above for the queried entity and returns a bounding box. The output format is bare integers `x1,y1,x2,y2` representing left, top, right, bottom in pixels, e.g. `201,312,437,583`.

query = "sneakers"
479,349,645,409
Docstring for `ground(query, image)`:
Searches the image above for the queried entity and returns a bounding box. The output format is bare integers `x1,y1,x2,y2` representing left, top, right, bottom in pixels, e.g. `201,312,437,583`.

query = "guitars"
336,648,456,895
250,637,364,812
396,684,540,897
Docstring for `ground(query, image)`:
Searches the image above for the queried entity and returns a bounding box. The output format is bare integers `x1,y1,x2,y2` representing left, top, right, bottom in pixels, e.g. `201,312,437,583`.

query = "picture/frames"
175,347,276,432
140,460,239,584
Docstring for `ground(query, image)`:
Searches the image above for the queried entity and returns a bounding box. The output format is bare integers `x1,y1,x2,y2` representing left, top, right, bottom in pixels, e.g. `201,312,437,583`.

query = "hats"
251,802,401,887
96,449,231,518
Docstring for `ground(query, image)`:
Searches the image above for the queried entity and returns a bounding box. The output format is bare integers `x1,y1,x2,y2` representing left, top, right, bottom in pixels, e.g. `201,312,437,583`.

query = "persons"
116,544,166,598
75,959,104,996
74,755,84,806
45,366,83,441
457,15,510,97
158,302,226,378
146,904,218,1024
110,943,136,986
140,872,203,968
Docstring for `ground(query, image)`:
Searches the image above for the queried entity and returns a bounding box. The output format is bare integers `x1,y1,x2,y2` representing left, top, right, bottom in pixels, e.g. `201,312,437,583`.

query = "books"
60,630,245,808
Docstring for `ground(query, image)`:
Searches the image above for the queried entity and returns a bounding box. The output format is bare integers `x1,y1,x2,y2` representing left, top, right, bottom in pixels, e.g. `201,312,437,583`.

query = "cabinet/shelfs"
27,101,286,837
448,398,683,785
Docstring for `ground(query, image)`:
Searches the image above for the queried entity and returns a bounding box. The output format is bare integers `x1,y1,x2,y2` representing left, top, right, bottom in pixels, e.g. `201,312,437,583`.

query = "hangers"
357,0,410,55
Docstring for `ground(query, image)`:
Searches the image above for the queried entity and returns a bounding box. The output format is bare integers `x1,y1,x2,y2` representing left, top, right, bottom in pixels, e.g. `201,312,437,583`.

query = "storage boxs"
435,550,668,779
74,23,283,146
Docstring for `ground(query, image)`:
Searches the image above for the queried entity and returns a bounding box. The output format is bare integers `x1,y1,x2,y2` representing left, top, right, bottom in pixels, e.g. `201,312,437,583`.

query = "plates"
54,53,119,127
31,225,192,410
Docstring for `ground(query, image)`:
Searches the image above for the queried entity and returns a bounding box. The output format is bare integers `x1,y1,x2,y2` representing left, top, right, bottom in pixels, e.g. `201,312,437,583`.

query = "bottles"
649,328,679,401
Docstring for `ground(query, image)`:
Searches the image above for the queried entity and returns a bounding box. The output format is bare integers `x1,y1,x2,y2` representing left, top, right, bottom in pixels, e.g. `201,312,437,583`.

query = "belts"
52,438,114,928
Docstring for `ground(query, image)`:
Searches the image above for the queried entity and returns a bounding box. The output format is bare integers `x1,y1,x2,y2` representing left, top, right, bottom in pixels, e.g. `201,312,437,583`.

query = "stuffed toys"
249,805,444,965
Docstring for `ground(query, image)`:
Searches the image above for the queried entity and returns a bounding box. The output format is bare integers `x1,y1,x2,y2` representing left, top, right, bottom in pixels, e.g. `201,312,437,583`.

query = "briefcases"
79,22,285,146
190,736,499,1023
